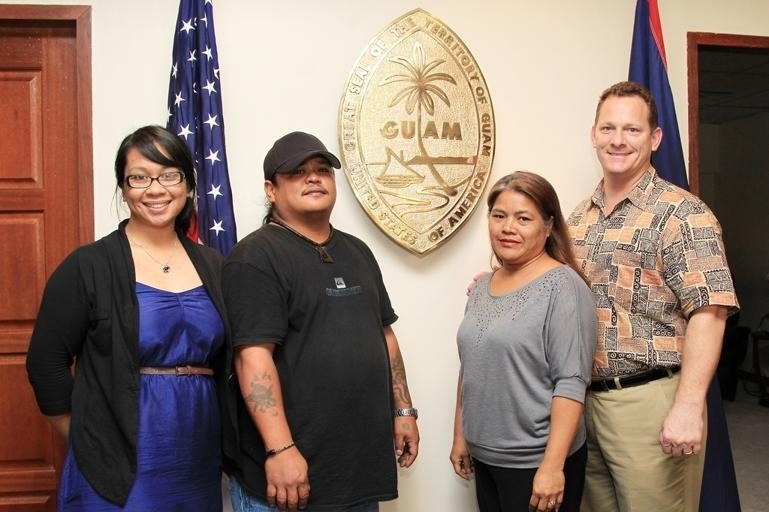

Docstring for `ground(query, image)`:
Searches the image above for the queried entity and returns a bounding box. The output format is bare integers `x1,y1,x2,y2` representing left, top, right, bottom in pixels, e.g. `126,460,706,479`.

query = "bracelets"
263,440,296,460
392,406,419,420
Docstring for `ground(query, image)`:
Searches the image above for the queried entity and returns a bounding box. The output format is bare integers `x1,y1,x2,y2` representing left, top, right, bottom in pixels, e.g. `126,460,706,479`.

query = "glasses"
124,169,189,189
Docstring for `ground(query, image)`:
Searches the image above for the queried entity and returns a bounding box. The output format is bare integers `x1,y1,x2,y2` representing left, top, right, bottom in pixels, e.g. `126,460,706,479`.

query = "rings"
547,497,558,506
662,443,672,448
683,449,694,456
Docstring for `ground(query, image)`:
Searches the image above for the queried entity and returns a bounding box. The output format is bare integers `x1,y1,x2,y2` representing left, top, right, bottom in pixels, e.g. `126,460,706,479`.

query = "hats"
260,130,343,181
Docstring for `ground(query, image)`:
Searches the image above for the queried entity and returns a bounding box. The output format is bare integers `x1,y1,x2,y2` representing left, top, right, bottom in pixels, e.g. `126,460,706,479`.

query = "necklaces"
265,214,337,264
118,228,181,277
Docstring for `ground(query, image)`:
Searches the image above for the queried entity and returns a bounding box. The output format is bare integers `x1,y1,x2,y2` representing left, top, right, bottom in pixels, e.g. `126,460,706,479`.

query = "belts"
583,361,680,394
138,364,218,376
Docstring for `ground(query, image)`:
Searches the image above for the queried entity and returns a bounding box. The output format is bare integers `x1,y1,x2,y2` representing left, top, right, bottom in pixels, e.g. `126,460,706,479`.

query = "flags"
160,0,244,259
625,2,745,511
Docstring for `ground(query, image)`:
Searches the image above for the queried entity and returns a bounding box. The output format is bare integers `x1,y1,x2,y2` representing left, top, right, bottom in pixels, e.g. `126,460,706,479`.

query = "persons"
219,131,421,511
464,80,741,512
448,169,600,512
24,125,231,509
